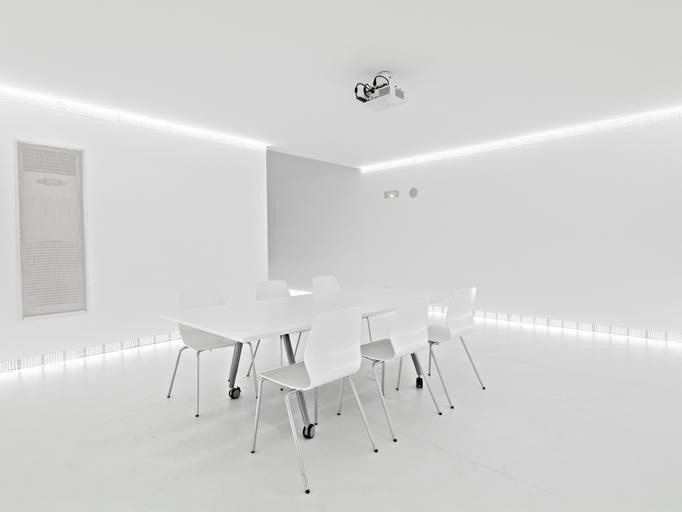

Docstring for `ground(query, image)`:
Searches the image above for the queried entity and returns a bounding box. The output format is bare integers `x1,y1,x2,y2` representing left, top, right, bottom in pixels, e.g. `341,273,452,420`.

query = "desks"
158,285,439,439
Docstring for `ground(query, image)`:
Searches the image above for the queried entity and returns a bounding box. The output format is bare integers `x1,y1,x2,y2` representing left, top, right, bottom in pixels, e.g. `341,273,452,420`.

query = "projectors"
362,84,408,112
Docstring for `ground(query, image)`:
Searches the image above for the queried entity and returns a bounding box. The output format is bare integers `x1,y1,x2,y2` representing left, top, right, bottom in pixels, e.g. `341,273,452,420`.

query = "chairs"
254,281,303,391
337,292,442,443
427,288,487,409
167,286,259,417
310,275,339,292
249,306,379,494
